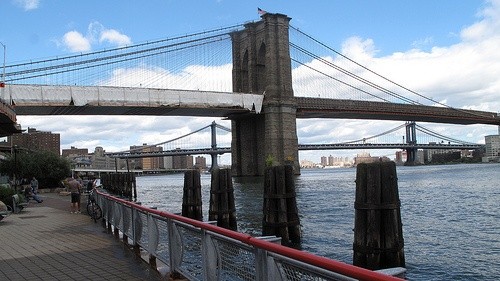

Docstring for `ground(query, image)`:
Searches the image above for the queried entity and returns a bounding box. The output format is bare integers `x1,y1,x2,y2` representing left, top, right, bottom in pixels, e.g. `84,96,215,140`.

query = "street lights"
12,144,21,195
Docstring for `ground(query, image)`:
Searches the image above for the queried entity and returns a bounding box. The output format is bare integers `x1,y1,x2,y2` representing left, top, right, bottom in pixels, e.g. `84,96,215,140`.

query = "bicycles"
81,185,103,224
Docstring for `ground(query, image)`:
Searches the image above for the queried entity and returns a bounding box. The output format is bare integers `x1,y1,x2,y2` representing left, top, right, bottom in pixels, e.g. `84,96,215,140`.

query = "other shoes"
38,199,43,203
69,211,77,214
78,211,81,213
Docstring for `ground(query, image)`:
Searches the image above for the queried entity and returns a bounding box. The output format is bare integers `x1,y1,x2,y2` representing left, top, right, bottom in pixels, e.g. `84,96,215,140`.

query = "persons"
86,178,96,191
70,174,82,214
30,176,38,194
25,187,43,203
